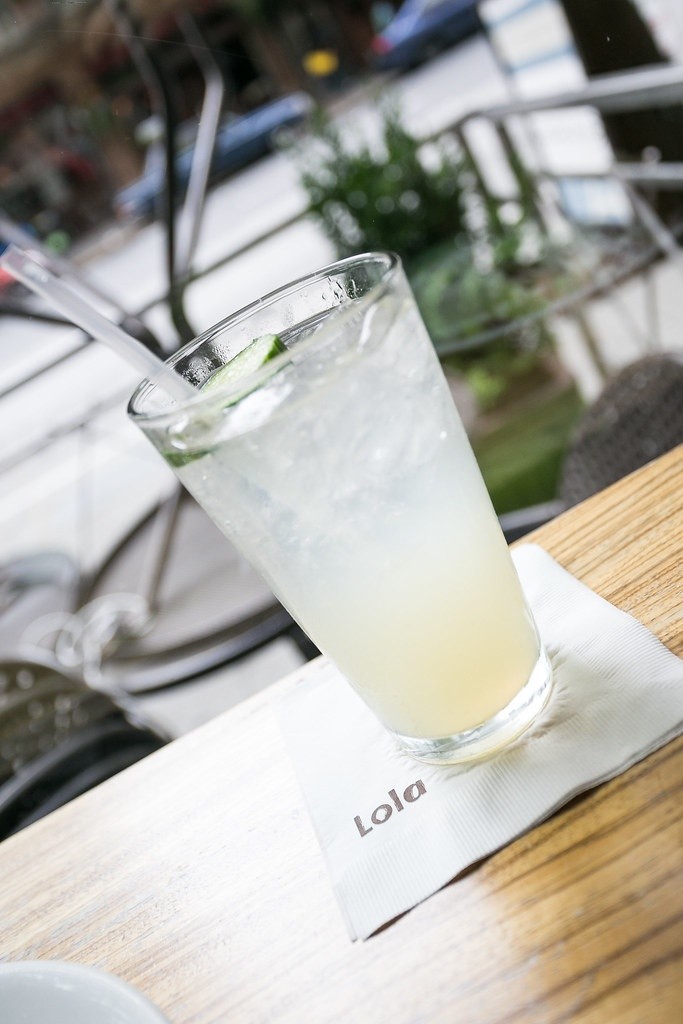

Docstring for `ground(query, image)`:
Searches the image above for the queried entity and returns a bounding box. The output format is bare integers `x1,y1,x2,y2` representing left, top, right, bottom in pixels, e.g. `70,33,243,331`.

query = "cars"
111,89,319,226
368,0,483,75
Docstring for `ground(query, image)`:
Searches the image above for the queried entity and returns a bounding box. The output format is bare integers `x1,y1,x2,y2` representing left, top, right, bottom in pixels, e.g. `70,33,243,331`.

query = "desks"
420,218,664,394
60,473,317,703
0,438,683,1024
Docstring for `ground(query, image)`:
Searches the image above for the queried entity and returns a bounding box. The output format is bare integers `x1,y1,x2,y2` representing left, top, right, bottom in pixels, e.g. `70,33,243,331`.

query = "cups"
0,960,173,1024
125,246,555,767
